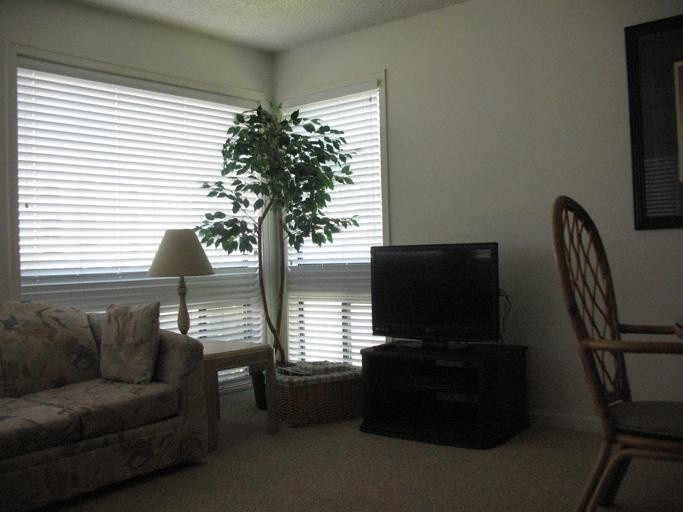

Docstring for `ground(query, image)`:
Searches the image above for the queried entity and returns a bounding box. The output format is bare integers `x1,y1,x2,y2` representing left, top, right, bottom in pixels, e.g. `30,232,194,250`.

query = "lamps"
147,229,213,335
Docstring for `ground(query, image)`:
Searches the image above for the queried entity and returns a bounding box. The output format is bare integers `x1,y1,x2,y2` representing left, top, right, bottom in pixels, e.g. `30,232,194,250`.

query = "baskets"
264,362,364,428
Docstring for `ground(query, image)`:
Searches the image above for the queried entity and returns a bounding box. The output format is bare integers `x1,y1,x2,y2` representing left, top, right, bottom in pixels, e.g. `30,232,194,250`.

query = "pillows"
99,304,160,384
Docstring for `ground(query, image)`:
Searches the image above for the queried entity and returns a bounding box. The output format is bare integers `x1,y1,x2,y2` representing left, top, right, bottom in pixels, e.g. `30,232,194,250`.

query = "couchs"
0,303,211,512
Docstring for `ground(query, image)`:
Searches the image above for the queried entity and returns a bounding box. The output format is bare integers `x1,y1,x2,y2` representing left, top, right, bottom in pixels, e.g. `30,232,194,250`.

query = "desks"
195,337,271,419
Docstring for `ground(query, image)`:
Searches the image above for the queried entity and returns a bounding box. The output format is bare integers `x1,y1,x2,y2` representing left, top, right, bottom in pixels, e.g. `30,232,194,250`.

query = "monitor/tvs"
369,241,502,352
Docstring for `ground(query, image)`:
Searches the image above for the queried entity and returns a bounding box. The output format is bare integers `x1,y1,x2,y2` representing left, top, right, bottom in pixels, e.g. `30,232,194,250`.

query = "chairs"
552,194,683,512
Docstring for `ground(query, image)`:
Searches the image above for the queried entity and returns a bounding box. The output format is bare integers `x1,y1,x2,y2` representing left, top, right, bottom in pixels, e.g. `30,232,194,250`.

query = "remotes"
375,344,397,352
